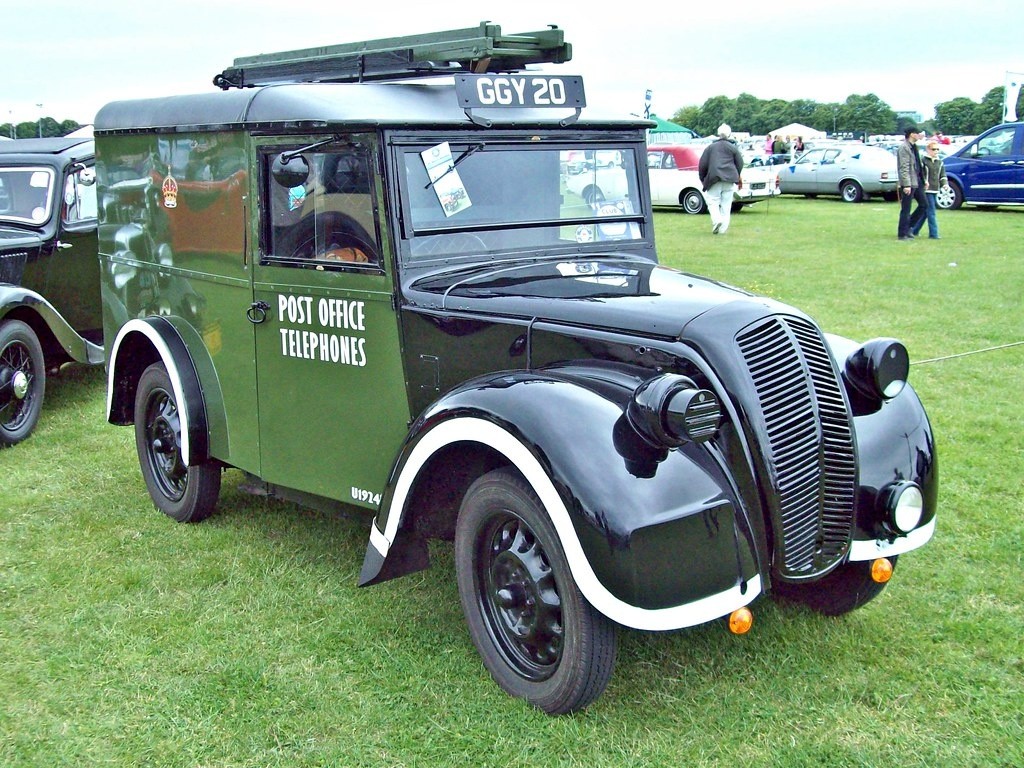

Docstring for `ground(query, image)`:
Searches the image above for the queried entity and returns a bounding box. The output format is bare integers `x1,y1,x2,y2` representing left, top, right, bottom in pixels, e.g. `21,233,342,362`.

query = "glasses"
929,148,939,151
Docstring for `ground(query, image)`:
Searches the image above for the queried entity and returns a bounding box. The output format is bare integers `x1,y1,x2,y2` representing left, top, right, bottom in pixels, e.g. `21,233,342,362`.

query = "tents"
644,112,701,144
768,122,827,139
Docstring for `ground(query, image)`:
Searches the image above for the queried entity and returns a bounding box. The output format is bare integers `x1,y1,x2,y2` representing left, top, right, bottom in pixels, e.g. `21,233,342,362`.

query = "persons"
895,128,928,239
919,129,952,145
912,142,948,242
698,123,744,235
794,136,804,158
747,144,755,150
763,134,792,163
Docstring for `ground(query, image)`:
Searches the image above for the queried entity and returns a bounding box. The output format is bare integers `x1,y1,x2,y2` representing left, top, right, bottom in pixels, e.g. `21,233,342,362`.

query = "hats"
904,127,922,134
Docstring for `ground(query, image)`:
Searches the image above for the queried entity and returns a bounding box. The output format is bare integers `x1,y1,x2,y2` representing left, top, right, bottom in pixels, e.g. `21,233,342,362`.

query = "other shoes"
713,222,721,234
907,230,915,238
898,236,911,240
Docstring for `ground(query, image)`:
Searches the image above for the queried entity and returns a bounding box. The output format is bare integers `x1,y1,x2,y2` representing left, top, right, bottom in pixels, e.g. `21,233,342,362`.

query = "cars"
779,145,902,202
647,142,781,216
934,122,1024,211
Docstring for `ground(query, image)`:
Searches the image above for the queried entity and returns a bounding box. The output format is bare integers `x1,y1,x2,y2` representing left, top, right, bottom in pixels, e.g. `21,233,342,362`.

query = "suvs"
92,22,944,717
0,133,105,446
